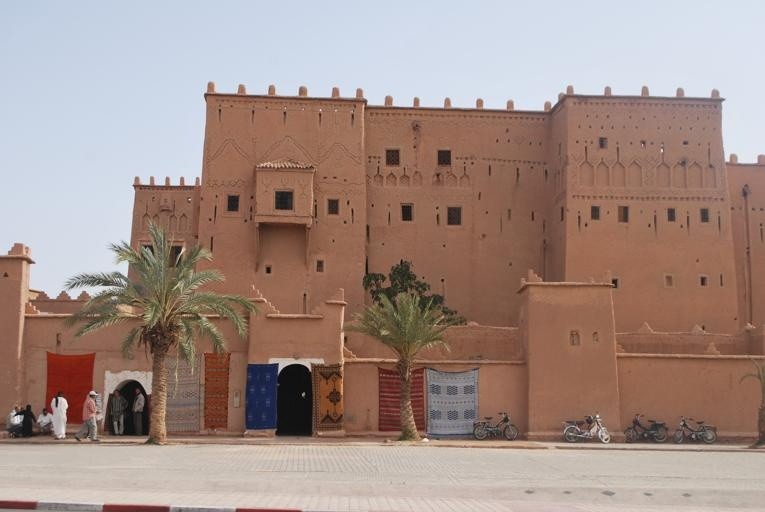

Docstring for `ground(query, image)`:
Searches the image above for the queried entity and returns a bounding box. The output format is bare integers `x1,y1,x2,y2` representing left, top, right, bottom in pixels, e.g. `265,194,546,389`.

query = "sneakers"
92,439,99,441
75,437,80,441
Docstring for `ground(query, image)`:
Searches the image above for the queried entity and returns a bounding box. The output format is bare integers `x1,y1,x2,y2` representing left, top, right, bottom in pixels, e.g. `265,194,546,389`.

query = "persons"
37,408,53,435
130,388,146,436
49,391,69,440
13,404,38,438
6,404,24,439
110,390,129,436
73,390,101,442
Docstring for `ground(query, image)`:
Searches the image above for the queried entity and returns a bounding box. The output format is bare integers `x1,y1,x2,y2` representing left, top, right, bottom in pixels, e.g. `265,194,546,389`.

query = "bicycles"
473,410,520,440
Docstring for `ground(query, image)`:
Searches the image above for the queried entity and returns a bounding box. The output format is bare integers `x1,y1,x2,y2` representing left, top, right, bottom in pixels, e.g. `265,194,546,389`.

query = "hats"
89,391,99,396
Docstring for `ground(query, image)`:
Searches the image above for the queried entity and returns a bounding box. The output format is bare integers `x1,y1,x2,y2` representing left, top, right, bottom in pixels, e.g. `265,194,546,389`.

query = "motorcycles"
673,415,719,444
563,411,611,443
624,414,668,442
